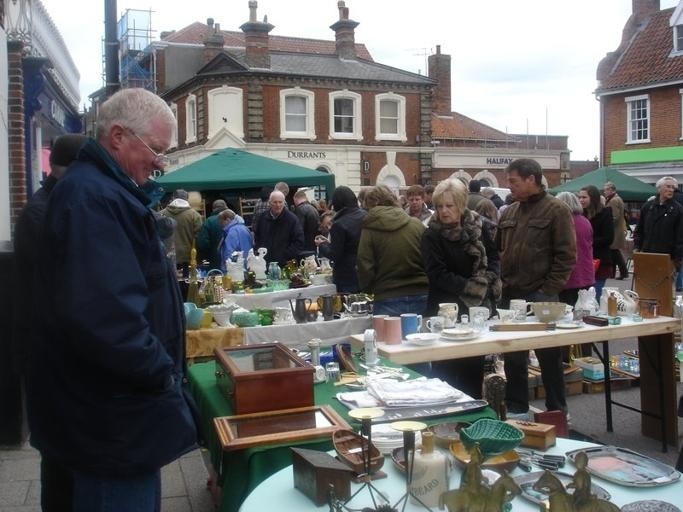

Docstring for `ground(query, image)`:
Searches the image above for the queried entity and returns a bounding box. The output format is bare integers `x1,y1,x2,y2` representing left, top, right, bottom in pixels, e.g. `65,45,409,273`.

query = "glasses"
112,120,171,169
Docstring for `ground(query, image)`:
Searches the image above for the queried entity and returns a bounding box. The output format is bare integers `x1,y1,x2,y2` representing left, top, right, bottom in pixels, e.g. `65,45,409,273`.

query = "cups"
325,362,340,383
371,303,459,345
496,308,519,323
468,306,489,336
510,299,533,321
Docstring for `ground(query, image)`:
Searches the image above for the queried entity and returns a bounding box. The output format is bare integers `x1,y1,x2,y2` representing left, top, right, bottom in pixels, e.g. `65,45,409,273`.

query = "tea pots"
288,293,311,323
342,300,374,318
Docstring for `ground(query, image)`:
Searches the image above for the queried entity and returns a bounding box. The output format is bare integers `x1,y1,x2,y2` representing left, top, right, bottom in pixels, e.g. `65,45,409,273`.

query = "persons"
23,86,203,510
12,131,92,511
158,174,682,349
418,176,501,403
354,182,429,374
493,160,580,421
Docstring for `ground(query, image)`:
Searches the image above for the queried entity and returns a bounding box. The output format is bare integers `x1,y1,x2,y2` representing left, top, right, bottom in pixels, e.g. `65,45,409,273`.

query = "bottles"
607,292,617,317
268,261,282,281
407,432,447,508
284,260,299,279
316,294,335,321
581,316,608,327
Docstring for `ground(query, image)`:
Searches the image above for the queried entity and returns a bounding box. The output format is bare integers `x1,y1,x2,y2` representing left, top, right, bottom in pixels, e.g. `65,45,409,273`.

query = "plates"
405,333,440,347
438,329,477,341
510,471,610,511
428,422,475,448
447,441,521,475
389,447,451,479
358,421,423,457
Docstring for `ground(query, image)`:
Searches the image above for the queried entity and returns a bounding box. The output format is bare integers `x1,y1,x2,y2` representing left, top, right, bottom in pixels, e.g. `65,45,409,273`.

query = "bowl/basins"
459,416,526,457
532,301,566,323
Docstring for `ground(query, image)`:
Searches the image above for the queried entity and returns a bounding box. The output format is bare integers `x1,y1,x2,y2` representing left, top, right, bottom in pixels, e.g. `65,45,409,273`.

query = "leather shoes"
615,273,628,280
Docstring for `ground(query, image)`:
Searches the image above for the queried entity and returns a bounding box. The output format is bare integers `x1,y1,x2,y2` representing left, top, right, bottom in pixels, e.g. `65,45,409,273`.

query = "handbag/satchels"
593,256,601,271
